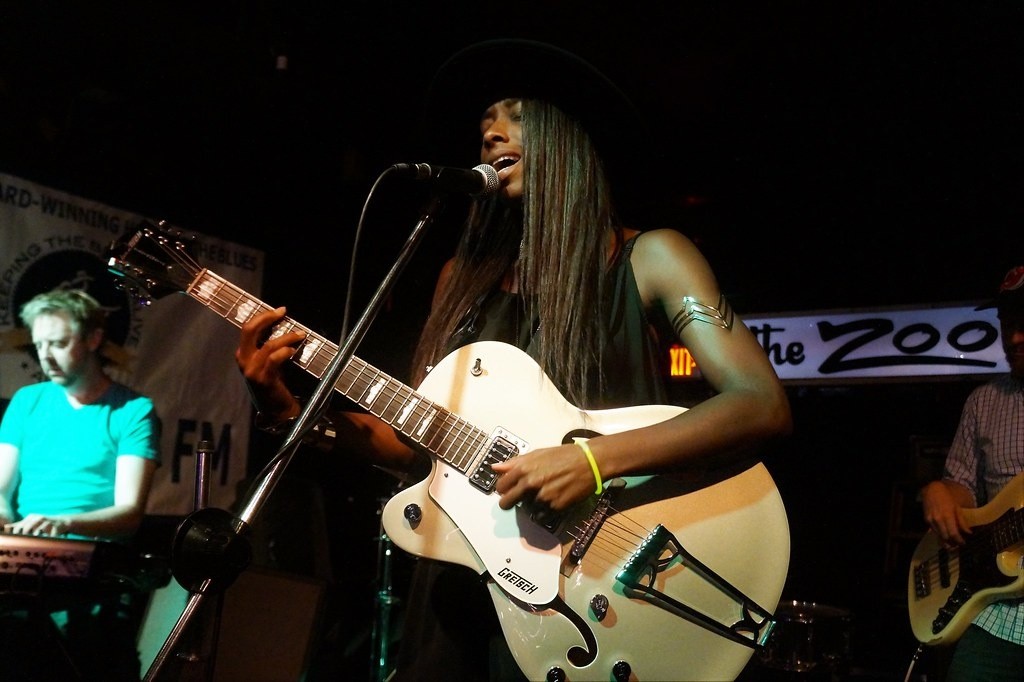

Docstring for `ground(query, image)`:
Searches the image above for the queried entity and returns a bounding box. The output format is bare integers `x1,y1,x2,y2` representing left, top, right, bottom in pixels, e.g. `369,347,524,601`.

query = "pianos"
0,523,120,586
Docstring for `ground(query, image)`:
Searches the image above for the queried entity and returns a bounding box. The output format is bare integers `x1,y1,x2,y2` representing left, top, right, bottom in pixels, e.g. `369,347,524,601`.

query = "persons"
235,39,795,681
1,291,162,627
921,252,1024,681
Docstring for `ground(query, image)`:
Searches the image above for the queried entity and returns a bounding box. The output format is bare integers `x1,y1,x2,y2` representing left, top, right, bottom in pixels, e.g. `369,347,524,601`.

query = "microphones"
395,163,499,197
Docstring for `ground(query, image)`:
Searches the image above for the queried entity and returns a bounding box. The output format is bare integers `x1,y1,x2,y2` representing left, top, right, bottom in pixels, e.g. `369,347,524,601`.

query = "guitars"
113,224,793,682
903,460,1024,650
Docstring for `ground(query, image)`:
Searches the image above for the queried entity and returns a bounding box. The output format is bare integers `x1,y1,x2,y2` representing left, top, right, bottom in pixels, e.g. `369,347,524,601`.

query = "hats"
423,38,623,147
974,266,1023,312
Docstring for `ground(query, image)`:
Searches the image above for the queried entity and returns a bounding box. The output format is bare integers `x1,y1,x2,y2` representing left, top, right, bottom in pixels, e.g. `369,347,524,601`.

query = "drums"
748,595,854,681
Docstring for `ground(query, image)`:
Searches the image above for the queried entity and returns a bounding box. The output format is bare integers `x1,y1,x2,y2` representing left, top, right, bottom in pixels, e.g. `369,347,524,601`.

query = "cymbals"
369,461,422,486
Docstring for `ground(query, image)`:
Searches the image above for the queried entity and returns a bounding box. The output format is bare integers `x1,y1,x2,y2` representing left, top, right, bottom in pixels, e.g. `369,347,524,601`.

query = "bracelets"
574,441,603,495
255,397,336,452
